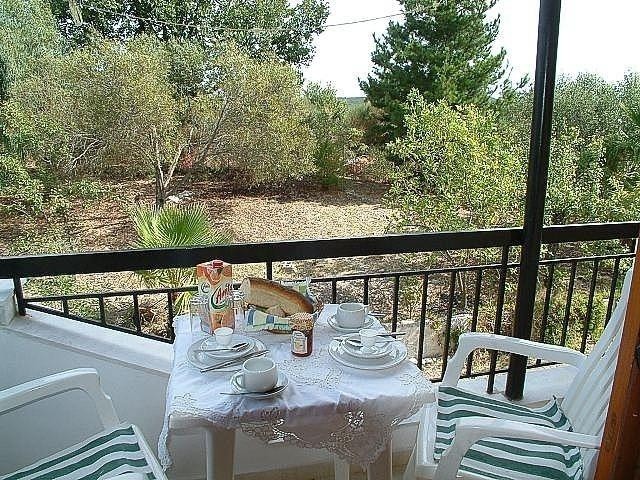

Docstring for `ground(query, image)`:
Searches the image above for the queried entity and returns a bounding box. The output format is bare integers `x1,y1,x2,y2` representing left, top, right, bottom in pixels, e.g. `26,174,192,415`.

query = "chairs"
402,268,632,480
0,367,170,479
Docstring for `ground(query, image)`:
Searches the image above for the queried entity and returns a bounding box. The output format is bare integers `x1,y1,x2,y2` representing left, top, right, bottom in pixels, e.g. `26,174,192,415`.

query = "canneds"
290,312,314,356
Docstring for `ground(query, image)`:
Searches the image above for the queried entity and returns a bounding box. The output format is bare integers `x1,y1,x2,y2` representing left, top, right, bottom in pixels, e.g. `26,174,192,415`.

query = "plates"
186,290,410,400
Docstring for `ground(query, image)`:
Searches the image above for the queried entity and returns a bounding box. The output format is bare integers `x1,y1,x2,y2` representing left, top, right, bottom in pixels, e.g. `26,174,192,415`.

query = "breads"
240,277,314,316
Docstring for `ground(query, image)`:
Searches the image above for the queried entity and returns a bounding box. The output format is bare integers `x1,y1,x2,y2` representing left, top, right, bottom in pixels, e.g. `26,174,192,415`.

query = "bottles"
195,258,236,333
